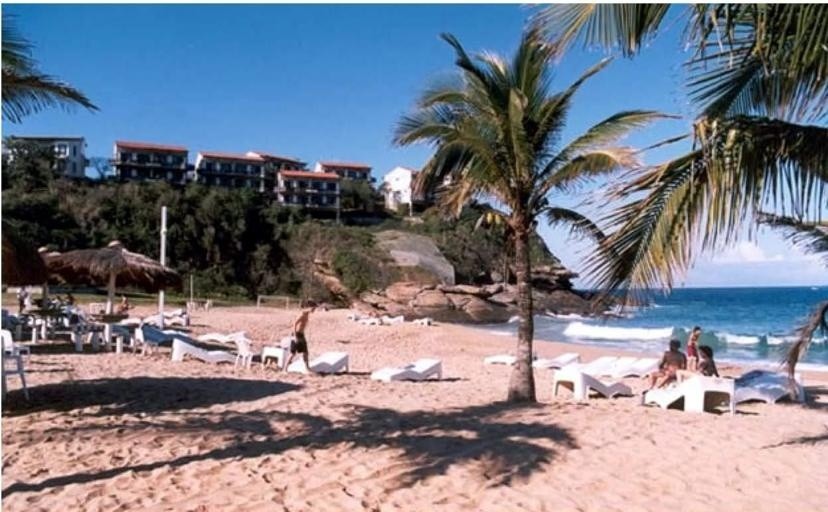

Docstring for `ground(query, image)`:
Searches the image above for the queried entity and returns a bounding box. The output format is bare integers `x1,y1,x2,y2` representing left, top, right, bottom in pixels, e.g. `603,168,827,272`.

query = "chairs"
551,370,632,401
2,328,31,404
1,308,193,356
168,330,440,383
487,352,664,377
733,370,805,404
348,311,435,328
641,373,737,414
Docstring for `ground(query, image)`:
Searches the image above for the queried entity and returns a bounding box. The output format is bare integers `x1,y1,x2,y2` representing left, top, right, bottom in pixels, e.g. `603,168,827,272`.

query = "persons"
282,300,319,373
686,326,702,372
641,339,686,395
672,345,720,388
15,285,131,315
